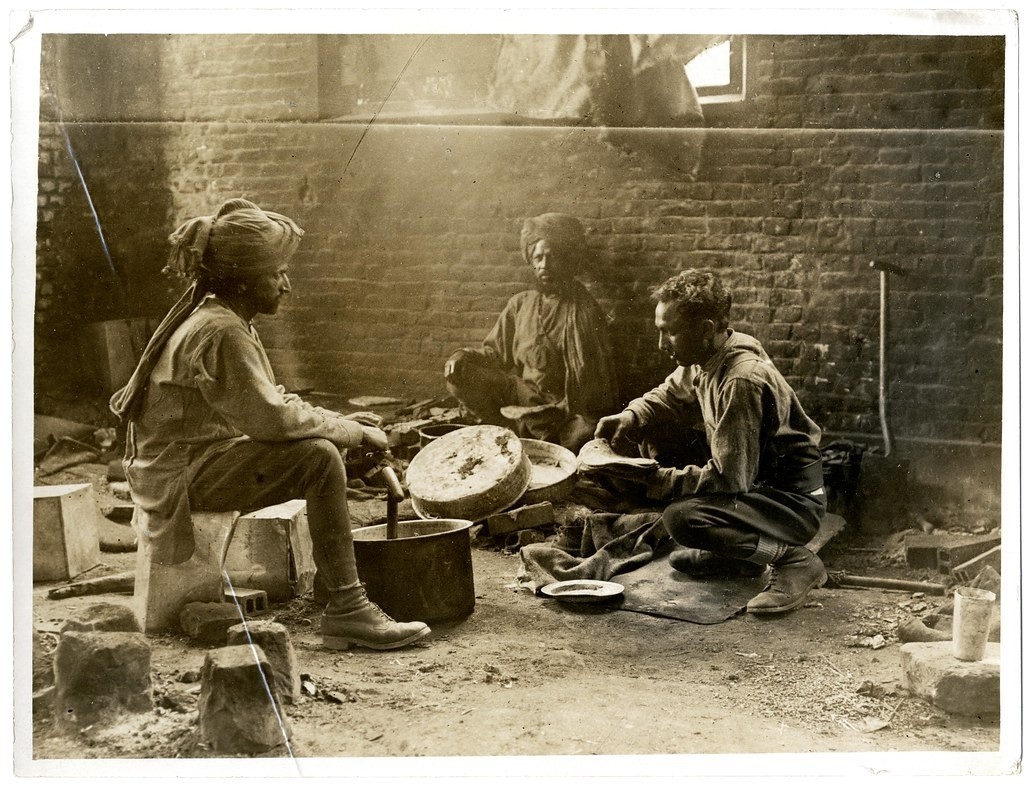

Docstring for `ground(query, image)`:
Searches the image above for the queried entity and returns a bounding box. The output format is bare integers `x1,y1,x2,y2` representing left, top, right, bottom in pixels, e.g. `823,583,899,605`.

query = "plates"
540,580,625,603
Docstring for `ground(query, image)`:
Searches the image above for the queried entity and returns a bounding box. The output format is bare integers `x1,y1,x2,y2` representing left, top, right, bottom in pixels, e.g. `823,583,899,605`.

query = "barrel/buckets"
351,518,475,620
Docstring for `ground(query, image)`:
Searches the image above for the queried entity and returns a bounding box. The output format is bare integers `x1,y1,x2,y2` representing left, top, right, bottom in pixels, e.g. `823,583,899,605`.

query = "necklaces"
535,291,564,344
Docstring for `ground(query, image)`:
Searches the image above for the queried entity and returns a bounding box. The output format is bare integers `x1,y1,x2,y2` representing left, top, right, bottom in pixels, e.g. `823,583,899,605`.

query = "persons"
445,211,622,455
109,198,433,651
595,268,828,615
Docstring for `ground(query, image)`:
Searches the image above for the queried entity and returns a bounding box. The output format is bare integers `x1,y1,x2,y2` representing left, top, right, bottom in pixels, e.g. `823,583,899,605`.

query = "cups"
953,587,998,661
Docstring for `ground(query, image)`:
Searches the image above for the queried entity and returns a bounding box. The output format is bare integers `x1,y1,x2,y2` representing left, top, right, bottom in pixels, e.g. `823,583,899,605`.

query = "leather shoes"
747,546,828,613
668,549,767,578
321,583,432,650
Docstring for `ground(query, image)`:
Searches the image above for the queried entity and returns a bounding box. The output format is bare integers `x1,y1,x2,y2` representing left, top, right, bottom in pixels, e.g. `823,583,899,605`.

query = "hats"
520,212,587,274
